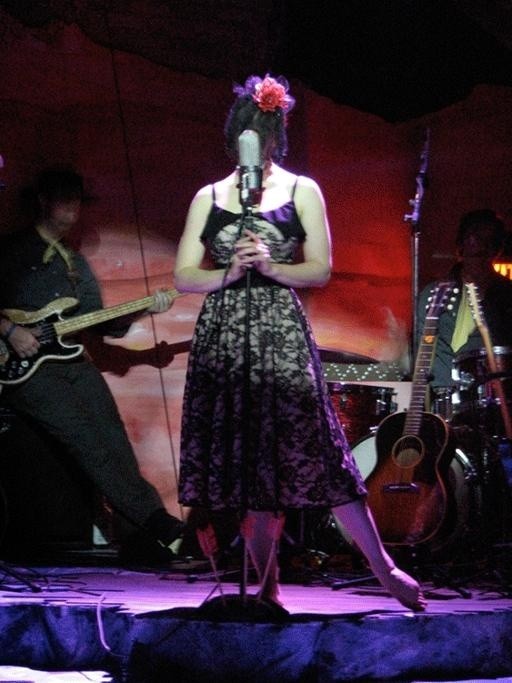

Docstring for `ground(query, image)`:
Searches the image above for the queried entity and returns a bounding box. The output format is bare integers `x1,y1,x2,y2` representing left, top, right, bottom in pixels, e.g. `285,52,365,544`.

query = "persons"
172,72,432,616
413,202,512,602
2,157,203,570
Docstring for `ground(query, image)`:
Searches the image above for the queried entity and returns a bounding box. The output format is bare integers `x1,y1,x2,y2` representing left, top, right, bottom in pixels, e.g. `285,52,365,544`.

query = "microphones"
238,129,262,205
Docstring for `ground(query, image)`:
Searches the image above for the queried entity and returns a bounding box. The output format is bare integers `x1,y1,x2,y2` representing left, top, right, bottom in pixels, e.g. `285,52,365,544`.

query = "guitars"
362,281,461,558
0,288,190,385
466,282,511,586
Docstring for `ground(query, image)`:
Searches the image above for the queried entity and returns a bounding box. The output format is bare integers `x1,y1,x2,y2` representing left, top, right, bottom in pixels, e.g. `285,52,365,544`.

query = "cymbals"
315,346,381,365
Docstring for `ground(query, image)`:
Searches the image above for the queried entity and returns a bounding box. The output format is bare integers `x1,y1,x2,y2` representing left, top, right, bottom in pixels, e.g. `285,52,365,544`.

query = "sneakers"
116,509,189,566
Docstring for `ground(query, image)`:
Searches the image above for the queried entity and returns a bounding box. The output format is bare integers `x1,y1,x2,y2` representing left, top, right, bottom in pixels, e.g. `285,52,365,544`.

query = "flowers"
251,75,288,112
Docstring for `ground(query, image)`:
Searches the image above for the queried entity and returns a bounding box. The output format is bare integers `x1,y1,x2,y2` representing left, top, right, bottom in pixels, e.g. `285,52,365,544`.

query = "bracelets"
3,320,17,342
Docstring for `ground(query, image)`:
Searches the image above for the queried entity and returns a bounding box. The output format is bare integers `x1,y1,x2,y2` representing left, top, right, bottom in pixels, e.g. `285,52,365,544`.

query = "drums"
330,431,482,563
327,382,393,443
